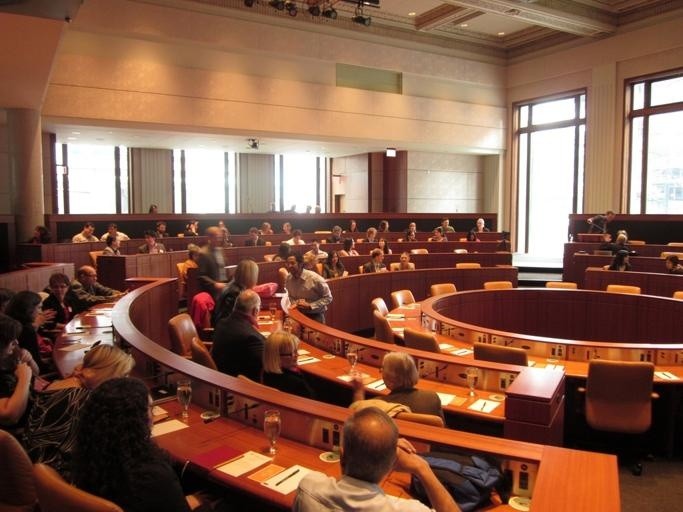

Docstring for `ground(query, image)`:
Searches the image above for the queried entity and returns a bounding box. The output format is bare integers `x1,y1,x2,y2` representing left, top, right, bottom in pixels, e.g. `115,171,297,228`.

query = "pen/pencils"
276,470,299,486
481,402,487,410
298,358,313,362
154,416,169,424
213,454,244,469
375,383,384,388
458,351,467,354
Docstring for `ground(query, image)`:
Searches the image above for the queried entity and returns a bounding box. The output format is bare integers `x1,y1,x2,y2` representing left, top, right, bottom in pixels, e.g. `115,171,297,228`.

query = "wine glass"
268,302,278,322
175,378,192,421
465,366,479,399
261,410,282,457
345,346,361,377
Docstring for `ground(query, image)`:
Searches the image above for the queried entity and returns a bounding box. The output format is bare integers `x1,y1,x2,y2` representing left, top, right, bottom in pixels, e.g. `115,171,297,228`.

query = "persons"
285,205,298,214
585,210,616,235
28,226,53,243
350,352,449,429
327,217,490,242
181,226,333,399
321,238,414,279
149,204,158,213
315,206,321,213
600,229,632,257
306,205,312,213
606,249,632,271
73,220,309,256
293,405,461,511
664,255,683,274
1,264,193,511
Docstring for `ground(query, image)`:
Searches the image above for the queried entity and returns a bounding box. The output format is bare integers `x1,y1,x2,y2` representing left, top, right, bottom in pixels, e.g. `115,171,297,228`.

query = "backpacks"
411,451,501,510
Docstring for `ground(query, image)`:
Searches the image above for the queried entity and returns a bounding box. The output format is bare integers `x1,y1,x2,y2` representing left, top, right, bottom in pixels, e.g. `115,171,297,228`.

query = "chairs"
0,212,682,512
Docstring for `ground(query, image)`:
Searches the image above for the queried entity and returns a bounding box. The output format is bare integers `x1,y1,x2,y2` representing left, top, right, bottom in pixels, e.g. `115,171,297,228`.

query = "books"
190,441,243,470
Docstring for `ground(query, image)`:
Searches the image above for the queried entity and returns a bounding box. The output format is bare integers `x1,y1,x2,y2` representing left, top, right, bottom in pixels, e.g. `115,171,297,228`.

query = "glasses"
278,351,296,358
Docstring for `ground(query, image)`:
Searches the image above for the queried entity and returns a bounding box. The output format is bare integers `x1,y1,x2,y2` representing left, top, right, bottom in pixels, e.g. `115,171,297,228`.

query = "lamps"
237,0,380,27
